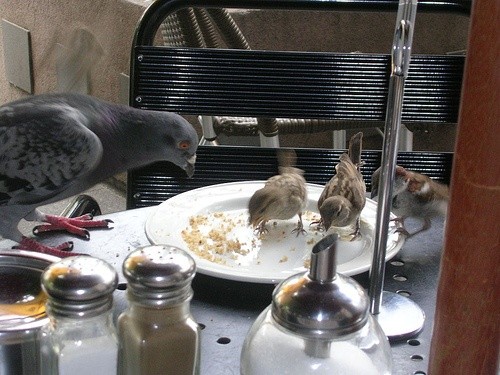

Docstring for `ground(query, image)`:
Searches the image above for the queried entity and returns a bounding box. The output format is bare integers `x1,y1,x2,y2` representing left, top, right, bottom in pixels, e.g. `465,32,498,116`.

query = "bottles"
34,255,124,375
117,244,200,375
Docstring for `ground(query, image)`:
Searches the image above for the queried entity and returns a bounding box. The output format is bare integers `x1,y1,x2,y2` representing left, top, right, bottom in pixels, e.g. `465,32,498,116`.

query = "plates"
145,180,405,284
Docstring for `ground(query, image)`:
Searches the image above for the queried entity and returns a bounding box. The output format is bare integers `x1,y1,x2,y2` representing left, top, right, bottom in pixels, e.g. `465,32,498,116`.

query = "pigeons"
1,91,199,257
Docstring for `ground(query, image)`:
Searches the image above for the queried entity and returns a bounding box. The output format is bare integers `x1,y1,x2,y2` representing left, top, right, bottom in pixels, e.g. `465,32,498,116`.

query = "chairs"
58,0,473,223
158,6,437,152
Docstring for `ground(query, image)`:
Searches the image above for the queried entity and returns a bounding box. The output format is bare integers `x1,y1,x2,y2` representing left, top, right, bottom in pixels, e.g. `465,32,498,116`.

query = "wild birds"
310,152,367,242
247,166,309,239
369,164,448,240
